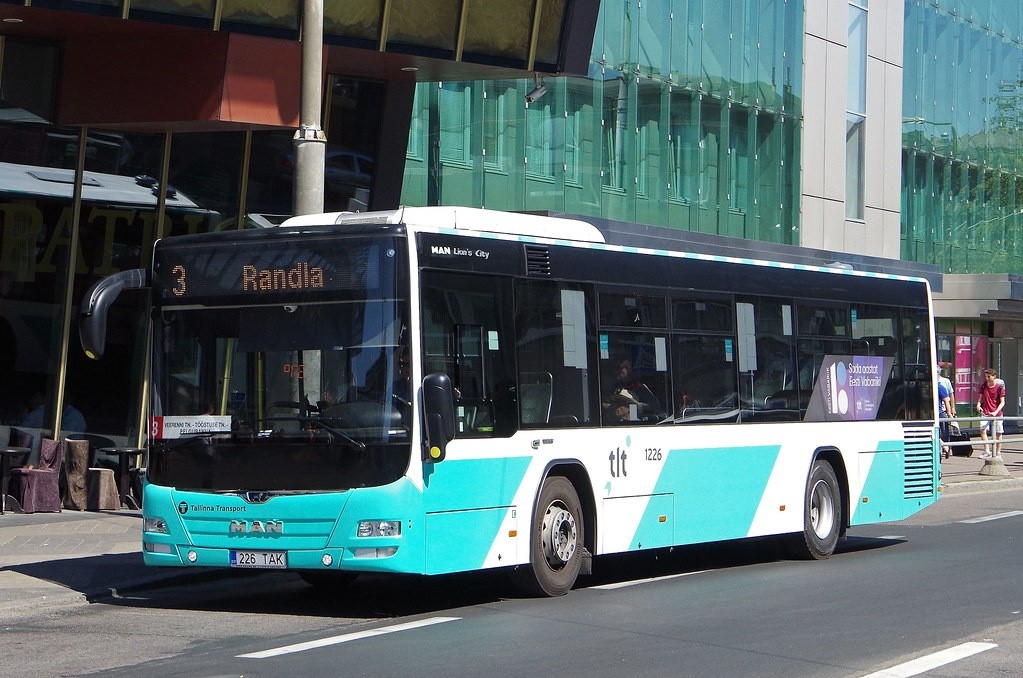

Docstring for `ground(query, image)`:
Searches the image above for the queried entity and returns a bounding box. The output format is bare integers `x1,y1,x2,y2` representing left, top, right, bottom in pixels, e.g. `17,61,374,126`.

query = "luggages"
949,425,973,458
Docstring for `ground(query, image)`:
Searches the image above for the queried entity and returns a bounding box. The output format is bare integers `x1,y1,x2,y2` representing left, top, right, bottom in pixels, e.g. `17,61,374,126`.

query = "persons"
937,365,957,459
600,352,668,424
322,348,461,410
976,368,1006,460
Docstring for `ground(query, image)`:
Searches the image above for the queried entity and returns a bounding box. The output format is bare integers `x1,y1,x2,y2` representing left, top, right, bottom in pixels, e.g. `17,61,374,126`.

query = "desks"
0,447,33,515
101,446,147,513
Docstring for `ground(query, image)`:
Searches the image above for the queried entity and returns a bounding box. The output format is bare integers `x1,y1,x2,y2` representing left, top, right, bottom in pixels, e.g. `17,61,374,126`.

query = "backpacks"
979,378,1005,402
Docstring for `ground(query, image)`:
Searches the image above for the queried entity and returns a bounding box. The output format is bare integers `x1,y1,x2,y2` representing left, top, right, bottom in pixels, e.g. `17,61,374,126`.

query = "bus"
79,205,946,597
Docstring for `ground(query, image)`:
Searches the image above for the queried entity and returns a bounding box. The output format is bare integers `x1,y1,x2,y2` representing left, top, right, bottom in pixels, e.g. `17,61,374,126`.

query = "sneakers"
978,451,992,459
944,445,951,460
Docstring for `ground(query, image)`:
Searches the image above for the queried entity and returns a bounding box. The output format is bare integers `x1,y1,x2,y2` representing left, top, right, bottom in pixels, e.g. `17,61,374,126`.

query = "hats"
608,388,643,410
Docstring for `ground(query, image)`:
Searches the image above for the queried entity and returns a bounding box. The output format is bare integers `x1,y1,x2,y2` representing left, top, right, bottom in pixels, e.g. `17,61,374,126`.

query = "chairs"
61,438,123,514
517,339,897,437
7,437,62,513
9,427,34,466
